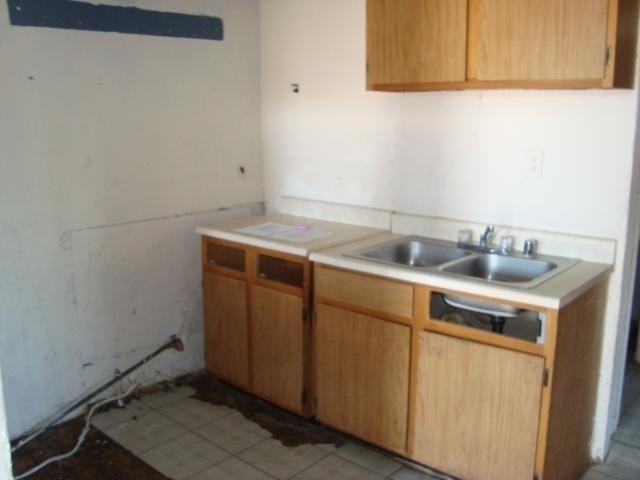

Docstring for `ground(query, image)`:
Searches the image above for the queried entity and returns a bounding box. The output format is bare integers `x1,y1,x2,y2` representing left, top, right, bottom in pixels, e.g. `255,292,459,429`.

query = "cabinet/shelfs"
311,260,611,480
201,234,314,419
364,0,640,93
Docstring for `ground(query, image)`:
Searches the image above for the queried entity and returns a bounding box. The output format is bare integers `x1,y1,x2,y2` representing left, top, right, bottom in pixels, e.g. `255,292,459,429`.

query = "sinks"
341,235,478,271
435,251,578,318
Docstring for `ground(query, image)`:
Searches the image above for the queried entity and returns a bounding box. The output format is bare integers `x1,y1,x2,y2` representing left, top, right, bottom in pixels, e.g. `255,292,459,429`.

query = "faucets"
481,224,493,249
